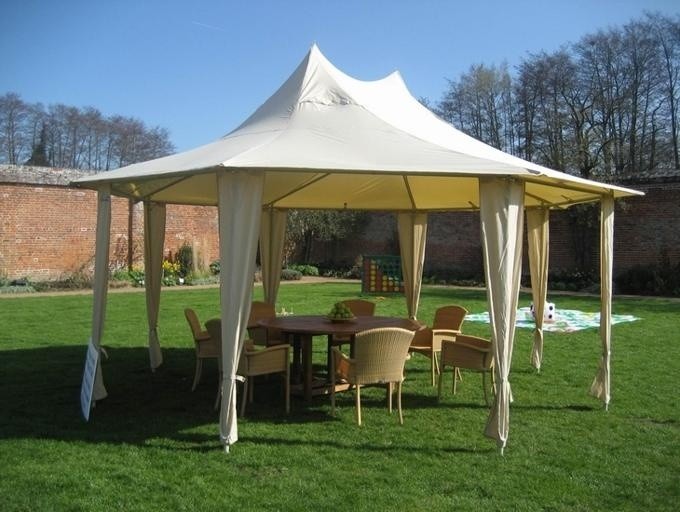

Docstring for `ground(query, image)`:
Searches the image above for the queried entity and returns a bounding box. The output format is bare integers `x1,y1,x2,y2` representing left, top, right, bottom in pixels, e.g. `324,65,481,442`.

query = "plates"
323,315,357,322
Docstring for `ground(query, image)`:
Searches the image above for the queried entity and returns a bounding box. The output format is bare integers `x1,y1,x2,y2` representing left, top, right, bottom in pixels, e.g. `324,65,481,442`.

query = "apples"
328,301,355,318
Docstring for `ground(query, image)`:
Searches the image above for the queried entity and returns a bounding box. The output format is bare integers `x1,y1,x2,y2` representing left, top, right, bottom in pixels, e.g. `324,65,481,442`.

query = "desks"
258,313,421,410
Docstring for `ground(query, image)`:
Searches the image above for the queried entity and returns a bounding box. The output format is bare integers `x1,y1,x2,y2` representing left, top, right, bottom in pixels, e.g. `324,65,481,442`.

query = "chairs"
408,305,468,386
438,331,497,403
183,308,252,394
204,317,293,417
247,300,276,320
330,326,416,425
336,299,376,317
